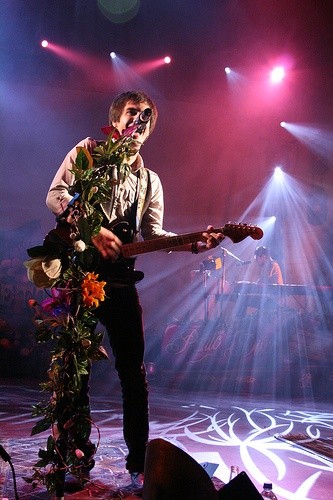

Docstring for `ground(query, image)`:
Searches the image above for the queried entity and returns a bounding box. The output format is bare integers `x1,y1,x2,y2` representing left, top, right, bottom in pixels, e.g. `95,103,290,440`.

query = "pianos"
233,280,333,295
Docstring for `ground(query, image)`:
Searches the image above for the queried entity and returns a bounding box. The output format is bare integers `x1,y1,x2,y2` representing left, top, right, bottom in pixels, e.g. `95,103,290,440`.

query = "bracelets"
191,242,198,254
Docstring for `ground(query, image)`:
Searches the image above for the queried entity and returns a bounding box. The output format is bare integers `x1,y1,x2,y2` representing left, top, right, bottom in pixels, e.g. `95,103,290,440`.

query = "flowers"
21,122,143,489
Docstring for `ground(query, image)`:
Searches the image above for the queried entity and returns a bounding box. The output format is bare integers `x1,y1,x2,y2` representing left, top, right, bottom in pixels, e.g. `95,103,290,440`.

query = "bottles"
260,482,278,500
230,466,239,482
150,362,154,375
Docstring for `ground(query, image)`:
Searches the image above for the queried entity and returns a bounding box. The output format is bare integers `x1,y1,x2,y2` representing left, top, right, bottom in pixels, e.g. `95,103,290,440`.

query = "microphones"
241,260,251,265
135,108,152,130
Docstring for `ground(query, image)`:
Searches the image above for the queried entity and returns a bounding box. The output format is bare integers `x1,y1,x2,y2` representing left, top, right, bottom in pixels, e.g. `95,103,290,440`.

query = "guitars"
40,220,264,288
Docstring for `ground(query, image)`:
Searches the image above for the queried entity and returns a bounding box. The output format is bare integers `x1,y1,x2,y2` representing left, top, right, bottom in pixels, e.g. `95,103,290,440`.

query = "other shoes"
63,469,89,489
130,471,144,488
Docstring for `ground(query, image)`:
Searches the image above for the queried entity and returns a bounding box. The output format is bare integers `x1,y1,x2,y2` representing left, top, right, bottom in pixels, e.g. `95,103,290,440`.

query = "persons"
46,89,225,489
254,245,283,284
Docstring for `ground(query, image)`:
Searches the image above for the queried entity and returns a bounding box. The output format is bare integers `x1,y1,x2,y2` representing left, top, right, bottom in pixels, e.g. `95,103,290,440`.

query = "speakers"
142,437,217,500
